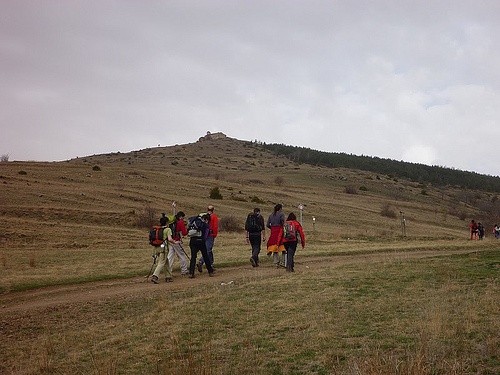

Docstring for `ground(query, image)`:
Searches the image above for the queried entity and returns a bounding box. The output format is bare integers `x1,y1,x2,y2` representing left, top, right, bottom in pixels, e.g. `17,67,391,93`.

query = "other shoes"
210,270,220,276
151,277,159,284
250,257,257,266
189,274,194,278
165,278,173,282
181,271,188,275
196,264,203,272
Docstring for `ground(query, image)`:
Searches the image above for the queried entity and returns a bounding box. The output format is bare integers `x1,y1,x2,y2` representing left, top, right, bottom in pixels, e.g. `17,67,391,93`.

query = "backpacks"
198,212,213,233
186,215,206,239
169,221,176,237
149,225,165,246
282,221,296,239
245,212,262,232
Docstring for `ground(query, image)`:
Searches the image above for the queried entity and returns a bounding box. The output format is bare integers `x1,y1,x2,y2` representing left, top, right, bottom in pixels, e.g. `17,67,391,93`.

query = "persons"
470,220,485,239
493,224,500,239
197,205,218,272
245,208,266,267
151,213,176,284
189,214,214,278
168,211,189,277
277,212,305,272
266,204,286,256
